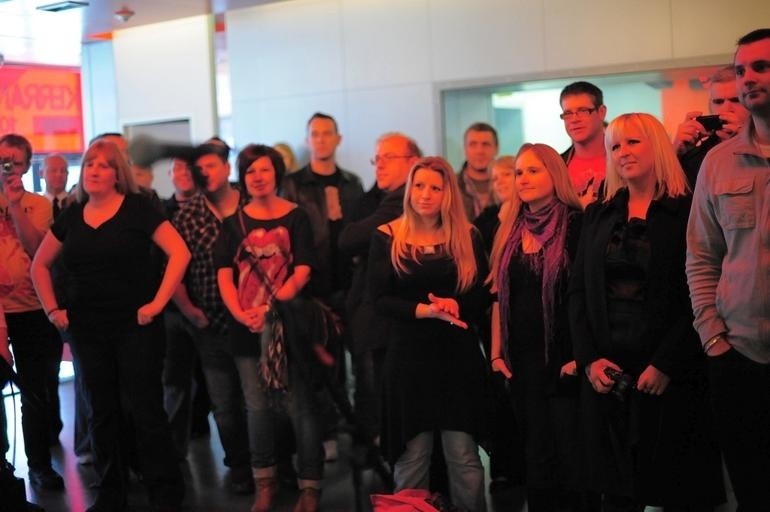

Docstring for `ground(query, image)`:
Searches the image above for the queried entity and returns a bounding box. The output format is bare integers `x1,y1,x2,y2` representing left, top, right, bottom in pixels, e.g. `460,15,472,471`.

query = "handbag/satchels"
273,298,340,361
370,489,458,512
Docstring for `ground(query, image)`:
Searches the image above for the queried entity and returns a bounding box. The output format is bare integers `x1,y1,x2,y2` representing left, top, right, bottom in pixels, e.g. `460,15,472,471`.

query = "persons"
0,28,770,512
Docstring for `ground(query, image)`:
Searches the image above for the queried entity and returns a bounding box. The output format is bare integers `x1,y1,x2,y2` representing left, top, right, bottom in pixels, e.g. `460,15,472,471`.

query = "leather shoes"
28,465,65,494
292,488,322,512
230,464,255,495
276,467,298,489
250,478,279,512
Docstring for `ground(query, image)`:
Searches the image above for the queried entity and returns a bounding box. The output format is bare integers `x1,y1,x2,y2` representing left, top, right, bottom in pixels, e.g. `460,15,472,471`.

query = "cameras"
696,115,723,130
0,159,15,174
605,371,636,402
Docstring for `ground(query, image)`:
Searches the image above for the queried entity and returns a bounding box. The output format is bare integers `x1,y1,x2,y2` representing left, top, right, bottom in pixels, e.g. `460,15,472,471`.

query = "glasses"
370,153,409,165
560,108,598,119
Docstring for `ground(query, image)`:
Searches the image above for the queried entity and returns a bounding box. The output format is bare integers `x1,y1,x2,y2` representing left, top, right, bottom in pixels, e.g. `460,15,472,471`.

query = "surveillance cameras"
115,15,130,23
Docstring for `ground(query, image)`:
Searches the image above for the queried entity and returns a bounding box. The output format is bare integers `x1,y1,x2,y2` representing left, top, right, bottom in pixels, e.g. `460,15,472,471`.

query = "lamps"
112,0,135,23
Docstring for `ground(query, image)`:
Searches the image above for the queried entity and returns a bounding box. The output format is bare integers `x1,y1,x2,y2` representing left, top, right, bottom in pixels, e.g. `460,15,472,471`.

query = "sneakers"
322,440,338,461
